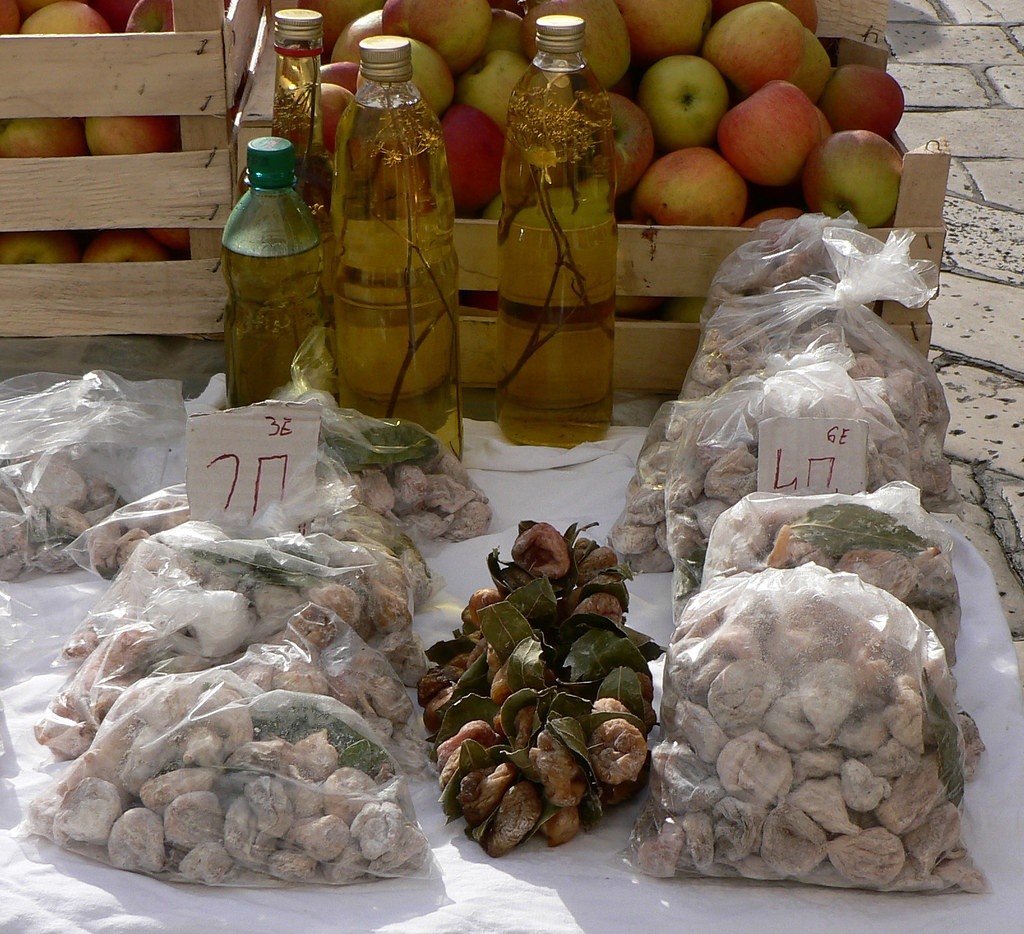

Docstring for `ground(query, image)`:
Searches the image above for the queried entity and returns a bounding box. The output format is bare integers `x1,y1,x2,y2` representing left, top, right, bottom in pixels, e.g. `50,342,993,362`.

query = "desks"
1,374,1024,933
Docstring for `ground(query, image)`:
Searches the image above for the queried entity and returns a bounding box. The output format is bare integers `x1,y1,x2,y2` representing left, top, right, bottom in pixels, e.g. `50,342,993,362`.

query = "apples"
0,0,192,265
287,0,906,331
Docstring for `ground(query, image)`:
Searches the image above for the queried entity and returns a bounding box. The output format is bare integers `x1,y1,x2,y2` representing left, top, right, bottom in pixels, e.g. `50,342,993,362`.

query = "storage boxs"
0,0,950,389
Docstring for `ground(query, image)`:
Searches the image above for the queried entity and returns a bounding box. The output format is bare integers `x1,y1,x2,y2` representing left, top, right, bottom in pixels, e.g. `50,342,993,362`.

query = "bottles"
239,9,335,406
495,15,616,446
333,36,462,464
218,135,324,410
